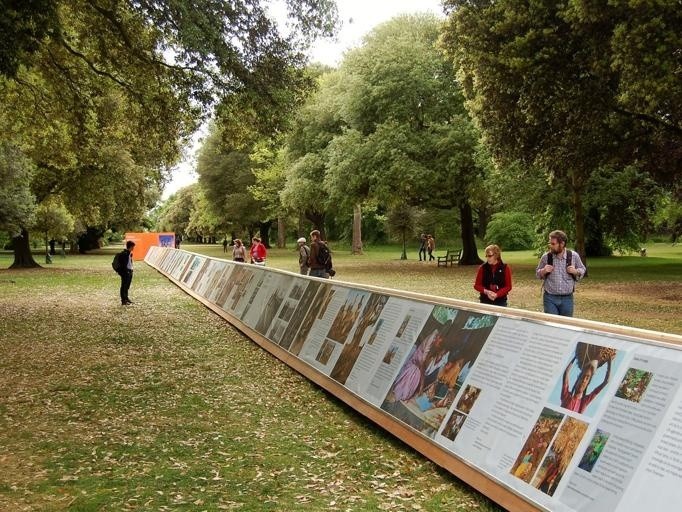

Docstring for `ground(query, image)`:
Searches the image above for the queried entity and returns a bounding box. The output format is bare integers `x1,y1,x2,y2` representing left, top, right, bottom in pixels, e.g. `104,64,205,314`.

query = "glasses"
551,242,555,245
485,254,494,257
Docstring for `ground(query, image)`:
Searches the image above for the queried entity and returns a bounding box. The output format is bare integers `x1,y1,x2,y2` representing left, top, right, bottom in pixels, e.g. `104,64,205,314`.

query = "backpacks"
315,242,331,265
112,251,130,275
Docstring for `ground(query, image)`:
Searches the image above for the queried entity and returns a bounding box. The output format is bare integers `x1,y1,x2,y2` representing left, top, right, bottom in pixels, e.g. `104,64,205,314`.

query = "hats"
297,237,306,242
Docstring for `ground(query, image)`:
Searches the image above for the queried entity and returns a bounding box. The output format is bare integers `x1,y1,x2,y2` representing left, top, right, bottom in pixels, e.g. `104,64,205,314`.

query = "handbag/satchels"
306,255,312,267
255,262,265,265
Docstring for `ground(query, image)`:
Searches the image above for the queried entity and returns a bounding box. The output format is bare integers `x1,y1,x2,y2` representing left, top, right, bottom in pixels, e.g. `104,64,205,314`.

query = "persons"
621,368,651,403
474,245,512,307
588,436,604,464
385,319,476,437
223,238,227,253
118,241,135,305
297,237,310,275
536,230,586,317
308,230,332,278
233,239,246,262
515,419,579,495
176,235,181,249
250,237,266,266
416,234,435,261
561,353,612,414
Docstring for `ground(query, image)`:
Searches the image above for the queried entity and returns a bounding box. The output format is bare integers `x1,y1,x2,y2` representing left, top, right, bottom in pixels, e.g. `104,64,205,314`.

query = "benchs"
437,249,462,268
